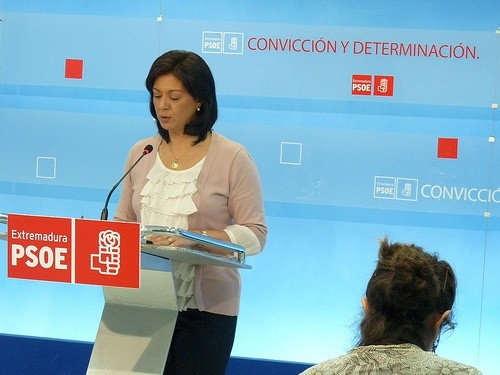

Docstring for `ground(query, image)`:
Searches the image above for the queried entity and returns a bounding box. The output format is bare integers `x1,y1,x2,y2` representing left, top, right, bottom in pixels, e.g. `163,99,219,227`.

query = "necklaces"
168,138,197,169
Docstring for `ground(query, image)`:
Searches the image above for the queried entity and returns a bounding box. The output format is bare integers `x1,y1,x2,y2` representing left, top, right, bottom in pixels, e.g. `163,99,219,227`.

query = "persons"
114,49,267,375
297,232,483,375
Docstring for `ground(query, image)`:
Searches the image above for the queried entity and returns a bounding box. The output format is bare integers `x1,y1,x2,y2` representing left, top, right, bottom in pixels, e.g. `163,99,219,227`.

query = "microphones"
101,144,153,220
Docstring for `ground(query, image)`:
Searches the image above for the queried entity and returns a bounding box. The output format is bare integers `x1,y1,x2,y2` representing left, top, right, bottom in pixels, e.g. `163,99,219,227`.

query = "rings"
168,237,173,242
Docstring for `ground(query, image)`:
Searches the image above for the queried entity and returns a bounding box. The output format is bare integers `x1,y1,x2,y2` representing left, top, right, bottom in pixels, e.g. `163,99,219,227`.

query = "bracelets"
200,229,208,237
